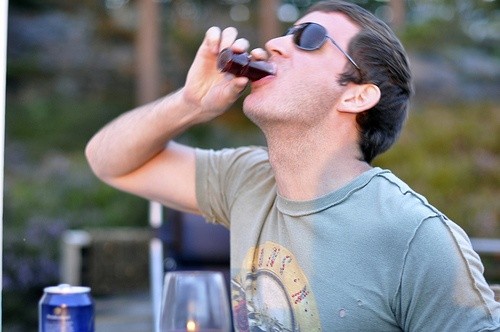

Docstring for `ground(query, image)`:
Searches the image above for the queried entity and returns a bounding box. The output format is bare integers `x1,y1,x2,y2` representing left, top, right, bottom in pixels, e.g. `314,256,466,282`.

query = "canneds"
36,284,96,332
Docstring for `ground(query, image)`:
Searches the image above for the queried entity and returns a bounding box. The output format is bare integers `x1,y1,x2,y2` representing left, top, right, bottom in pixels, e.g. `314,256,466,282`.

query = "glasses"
280,22,364,82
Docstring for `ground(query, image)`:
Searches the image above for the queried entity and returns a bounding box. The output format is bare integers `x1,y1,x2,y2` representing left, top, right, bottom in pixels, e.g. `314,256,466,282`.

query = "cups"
158,271,231,332
216,47,278,83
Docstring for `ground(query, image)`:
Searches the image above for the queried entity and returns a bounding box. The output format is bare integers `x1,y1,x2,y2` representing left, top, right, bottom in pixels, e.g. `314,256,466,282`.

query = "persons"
84,0,500,331
